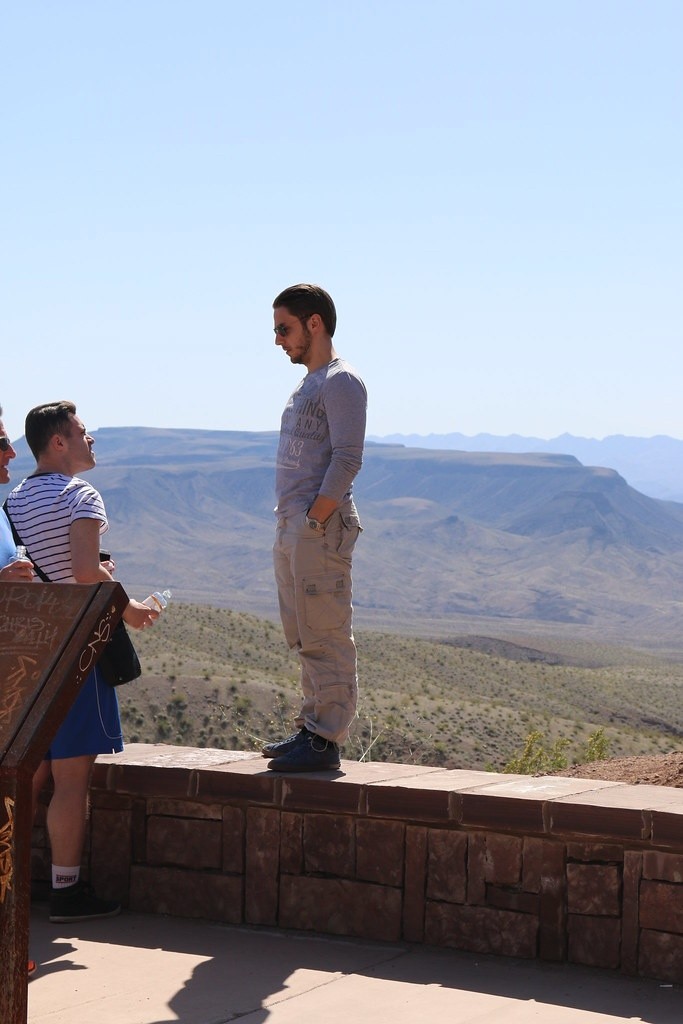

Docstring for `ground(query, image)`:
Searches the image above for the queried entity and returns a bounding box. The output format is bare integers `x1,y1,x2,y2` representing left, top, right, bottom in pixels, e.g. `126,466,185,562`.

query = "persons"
0,402,159,923
262,283,368,772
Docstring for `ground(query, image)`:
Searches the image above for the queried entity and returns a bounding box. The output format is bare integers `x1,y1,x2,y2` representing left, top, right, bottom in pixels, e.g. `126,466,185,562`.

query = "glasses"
0,437,10,451
274,316,310,337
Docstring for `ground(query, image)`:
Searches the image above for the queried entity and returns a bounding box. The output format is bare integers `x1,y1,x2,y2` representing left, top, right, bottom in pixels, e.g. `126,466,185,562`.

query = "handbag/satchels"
96,618,142,686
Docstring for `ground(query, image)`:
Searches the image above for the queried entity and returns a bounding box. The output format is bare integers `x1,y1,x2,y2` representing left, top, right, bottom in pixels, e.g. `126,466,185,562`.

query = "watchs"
305,516,324,530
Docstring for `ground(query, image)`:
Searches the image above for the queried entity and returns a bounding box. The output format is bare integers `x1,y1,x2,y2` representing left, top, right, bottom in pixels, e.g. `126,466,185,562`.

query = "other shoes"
27,960,37,974
49,881,122,922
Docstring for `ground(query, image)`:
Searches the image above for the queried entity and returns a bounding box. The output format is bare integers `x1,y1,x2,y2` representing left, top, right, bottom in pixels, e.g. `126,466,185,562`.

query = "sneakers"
268,731,341,771
262,726,307,757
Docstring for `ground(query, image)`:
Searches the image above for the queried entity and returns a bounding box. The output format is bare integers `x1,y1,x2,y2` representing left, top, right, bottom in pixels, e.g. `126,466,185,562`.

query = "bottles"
100,552,113,575
142,590,172,624
9,545,31,572
